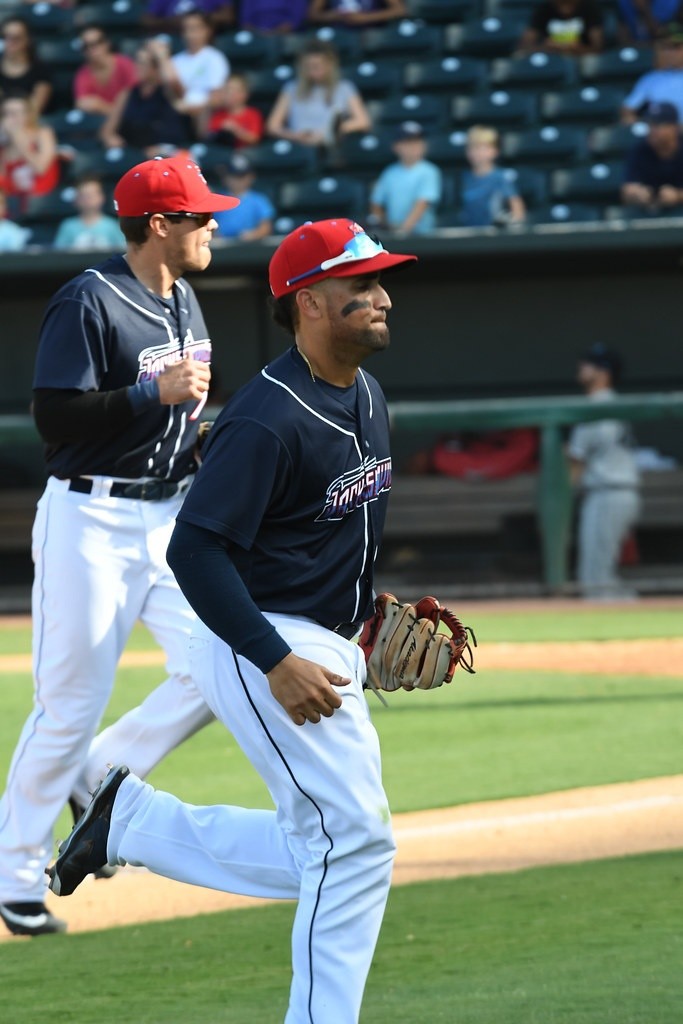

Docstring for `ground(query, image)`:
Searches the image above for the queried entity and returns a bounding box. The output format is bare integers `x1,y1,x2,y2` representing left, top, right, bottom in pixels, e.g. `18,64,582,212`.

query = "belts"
68,475,179,501
319,619,359,639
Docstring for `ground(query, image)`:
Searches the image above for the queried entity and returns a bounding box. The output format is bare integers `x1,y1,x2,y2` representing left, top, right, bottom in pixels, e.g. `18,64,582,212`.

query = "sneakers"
45,764,130,897
1,901,58,935
68,796,116,879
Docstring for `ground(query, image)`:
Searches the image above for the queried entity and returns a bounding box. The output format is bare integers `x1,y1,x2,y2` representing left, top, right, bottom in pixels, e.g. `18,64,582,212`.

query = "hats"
393,122,426,142
114,156,240,217
268,218,416,300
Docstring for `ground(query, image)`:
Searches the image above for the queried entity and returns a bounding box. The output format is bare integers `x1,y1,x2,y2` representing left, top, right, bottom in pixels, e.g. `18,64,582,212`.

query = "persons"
561,334,643,595
0,157,238,940
48,217,480,1023
0,0,683,252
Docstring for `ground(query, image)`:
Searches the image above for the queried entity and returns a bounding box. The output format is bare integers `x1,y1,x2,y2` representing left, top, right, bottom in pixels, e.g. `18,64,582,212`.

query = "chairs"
0,0,656,249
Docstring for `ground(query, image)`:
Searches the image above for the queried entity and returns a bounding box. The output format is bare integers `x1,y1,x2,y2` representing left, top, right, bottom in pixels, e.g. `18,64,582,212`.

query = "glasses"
286,233,388,286
143,212,213,227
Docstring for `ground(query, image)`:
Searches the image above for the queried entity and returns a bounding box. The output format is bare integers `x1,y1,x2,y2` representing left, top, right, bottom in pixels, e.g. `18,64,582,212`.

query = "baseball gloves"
366,589,478,693
